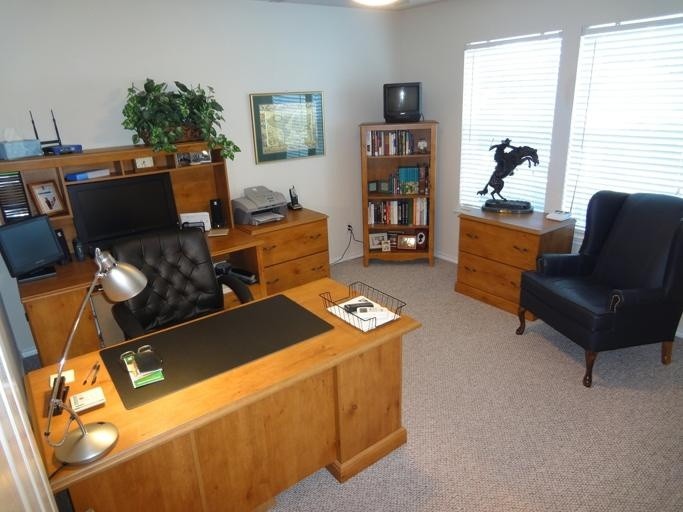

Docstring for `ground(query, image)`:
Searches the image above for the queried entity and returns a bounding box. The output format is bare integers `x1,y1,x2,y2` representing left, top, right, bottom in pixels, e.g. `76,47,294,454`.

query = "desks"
7,223,268,368
20,273,424,509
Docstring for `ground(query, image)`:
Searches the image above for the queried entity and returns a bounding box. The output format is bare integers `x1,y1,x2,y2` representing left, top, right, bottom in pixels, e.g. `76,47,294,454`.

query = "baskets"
319,281,406,333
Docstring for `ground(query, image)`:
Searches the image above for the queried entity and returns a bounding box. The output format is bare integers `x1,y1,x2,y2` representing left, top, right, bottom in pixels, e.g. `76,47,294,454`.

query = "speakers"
210,198,225,228
53,229,72,265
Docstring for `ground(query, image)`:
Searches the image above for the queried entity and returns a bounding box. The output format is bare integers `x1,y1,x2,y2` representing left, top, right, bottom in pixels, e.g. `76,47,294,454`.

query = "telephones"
287,185,302,210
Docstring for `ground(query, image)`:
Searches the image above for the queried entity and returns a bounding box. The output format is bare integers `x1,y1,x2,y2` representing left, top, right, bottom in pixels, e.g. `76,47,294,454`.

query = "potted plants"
116,73,244,164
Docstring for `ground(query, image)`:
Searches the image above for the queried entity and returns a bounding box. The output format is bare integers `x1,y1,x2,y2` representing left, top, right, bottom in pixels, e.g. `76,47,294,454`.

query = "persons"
489,138,519,164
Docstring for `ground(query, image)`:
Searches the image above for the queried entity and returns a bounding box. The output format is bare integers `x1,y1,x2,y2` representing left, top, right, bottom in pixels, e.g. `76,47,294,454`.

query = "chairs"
100,224,257,341
509,186,683,389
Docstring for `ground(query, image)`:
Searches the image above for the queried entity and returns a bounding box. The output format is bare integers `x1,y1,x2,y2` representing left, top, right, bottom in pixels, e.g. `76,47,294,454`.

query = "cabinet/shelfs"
0,135,235,274
451,206,576,323
234,197,331,298
357,116,441,269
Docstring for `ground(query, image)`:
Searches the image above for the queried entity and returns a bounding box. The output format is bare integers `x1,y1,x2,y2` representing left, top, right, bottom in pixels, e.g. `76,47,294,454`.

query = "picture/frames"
24,178,68,221
248,88,326,167
185,149,212,167
367,229,418,252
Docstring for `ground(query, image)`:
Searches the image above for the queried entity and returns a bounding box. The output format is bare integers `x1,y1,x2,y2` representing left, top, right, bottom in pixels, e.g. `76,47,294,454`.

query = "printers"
231,185,288,226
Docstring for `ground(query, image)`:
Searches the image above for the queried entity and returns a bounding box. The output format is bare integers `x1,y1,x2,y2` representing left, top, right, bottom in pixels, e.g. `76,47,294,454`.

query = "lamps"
37,245,153,473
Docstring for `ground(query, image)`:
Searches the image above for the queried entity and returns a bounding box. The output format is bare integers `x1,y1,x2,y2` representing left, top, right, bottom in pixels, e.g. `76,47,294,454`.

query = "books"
366,131,429,248
123,354,165,389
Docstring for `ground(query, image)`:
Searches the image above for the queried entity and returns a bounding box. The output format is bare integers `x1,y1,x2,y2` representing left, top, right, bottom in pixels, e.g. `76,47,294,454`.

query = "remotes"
344,301,373,313
357,307,388,317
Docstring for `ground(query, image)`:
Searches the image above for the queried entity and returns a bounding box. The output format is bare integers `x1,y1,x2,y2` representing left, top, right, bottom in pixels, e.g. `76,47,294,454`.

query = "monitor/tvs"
67,171,180,259
0,214,64,282
383,82,421,123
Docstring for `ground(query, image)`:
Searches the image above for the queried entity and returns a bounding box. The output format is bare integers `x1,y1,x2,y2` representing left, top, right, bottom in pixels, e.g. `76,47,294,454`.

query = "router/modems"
29,109,82,156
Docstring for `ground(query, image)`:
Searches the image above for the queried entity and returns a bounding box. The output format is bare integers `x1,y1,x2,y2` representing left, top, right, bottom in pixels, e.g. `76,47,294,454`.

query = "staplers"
49,376,69,415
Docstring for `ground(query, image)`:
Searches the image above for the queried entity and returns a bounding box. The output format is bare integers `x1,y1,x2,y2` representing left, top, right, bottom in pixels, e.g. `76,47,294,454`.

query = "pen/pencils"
83,361,100,385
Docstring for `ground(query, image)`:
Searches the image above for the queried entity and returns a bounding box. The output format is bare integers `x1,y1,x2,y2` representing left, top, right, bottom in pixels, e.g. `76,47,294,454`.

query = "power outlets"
345,224,354,233
